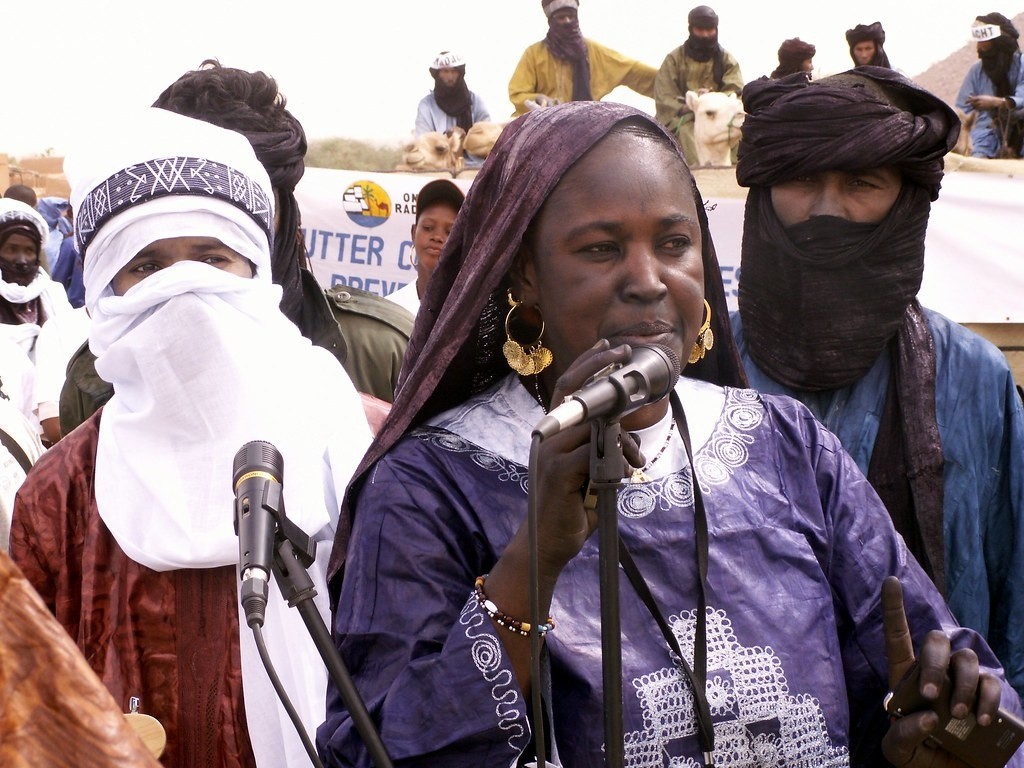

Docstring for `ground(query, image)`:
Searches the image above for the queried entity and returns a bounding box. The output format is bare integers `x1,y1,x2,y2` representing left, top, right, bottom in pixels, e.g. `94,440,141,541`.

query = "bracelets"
474,572,554,638
1002,98,1005,106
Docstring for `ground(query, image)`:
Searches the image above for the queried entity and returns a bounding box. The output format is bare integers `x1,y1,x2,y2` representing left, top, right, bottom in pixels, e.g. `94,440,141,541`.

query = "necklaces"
535,375,675,484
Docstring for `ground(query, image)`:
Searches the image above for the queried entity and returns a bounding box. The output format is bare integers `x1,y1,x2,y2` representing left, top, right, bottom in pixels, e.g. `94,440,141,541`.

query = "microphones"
230,440,284,631
532,342,680,444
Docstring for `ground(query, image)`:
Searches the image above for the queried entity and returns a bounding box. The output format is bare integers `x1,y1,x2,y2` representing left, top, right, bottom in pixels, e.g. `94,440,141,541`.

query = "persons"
508,0,660,118
772,38,816,80
654,5,744,167
845,21,891,70
317,100,1024,768
58,58,416,433
7,107,392,768
385,178,466,313
416,44,491,167
956,12,1024,159
0,184,92,551
728,64,1024,701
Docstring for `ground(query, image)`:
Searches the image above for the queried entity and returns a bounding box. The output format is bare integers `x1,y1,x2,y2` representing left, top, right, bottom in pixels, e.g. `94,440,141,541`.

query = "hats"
977,11,1020,42
541,0,580,14
0,196,49,250
777,37,817,64
735,65,956,202
689,6,721,31
62,105,281,303
414,176,466,214
845,21,887,46
429,51,467,78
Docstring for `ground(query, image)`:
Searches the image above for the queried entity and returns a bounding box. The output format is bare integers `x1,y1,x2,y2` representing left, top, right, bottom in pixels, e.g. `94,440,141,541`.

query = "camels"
395,115,512,175
685,91,749,200
950,108,1024,181
